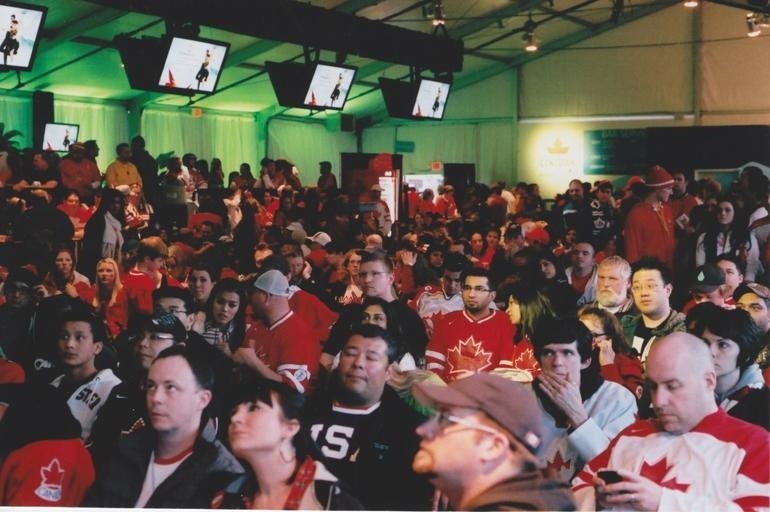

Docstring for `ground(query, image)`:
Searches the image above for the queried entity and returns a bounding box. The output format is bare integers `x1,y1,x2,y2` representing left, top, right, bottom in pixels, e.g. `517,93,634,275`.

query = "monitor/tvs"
114,35,195,97
378,76,424,121
0,1,48,72
42,121,80,154
409,78,452,121
302,60,358,111
265,59,326,111
154,32,231,96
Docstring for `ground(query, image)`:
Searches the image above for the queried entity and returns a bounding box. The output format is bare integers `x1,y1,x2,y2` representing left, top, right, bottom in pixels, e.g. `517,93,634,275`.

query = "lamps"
432,0,445,26
524,30,538,51
746,12,770,37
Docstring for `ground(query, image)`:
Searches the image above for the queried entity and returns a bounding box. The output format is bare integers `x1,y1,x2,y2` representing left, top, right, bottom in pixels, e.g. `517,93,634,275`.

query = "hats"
151,313,185,339
645,166,675,189
287,221,331,246
304,250,328,268
622,176,646,190
366,234,382,249
505,223,550,244
411,375,542,456
252,269,291,296
689,264,725,293
734,282,770,302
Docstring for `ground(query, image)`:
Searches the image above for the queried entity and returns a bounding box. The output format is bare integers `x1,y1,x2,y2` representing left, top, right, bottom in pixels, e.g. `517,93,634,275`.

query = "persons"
192,377,367,509
63,128,71,151
432,86,442,117
74,345,246,508
290,322,438,510
195,50,211,91
569,331,770,512
329,72,343,107
0,14,20,65
183,151,769,423
0,135,191,509
410,372,579,511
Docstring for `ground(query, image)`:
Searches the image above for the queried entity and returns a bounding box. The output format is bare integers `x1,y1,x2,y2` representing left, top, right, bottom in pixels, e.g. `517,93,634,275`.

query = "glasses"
423,405,510,448
592,333,607,338
463,286,493,293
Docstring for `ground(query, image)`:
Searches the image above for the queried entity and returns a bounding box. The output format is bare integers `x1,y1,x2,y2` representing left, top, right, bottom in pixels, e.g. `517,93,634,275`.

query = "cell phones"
597,468,632,507
